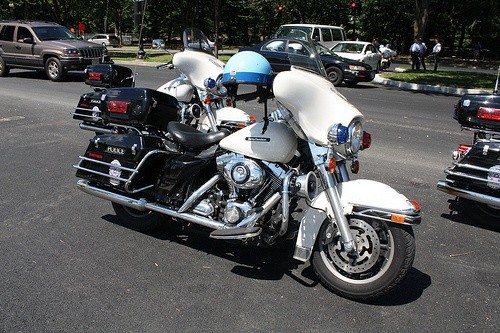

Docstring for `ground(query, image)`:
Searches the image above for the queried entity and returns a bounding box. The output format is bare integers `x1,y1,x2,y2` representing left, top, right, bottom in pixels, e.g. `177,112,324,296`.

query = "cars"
238,37,374,88
87,33,120,48
326,41,383,74
187,35,215,54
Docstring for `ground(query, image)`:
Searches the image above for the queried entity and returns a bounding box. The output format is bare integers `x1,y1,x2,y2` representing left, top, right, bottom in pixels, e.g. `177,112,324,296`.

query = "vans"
273,23,348,52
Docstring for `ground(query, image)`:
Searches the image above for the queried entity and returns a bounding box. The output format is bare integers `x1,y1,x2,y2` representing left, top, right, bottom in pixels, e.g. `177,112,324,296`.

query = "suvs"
0,18,115,83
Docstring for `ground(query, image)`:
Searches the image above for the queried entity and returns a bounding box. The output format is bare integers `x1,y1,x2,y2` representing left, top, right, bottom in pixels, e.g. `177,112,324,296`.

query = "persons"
410,38,427,70
433,39,441,71
383,44,391,58
473,42,481,59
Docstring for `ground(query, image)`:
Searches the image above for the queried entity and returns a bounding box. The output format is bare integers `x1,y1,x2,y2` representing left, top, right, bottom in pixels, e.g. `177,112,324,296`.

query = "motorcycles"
434,88,500,232
68,26,422,303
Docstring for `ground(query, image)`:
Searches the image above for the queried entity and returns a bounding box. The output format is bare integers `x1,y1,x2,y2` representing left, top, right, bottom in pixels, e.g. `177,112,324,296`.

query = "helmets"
222,51,274,94
